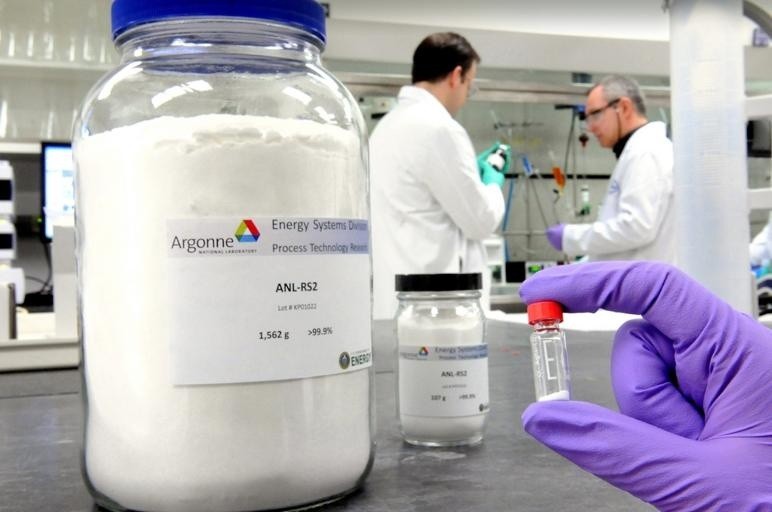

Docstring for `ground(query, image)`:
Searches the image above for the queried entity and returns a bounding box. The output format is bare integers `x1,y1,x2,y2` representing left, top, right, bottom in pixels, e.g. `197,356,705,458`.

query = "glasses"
583,98,620,123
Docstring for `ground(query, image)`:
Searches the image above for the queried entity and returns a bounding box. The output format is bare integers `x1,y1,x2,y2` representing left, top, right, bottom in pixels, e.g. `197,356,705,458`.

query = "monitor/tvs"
38,142,75,242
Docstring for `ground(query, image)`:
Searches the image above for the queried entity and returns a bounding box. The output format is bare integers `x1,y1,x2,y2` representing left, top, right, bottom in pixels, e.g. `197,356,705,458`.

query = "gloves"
477,141,511,186
546,224,563,251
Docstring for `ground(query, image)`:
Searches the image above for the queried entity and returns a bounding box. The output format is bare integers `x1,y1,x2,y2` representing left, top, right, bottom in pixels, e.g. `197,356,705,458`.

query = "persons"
368,32,510,321
546,73,675,265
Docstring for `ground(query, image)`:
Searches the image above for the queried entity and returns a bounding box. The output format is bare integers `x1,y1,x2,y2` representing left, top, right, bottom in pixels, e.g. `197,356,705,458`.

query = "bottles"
484,141,509,172
524,299,571,403
71,2,375,504
518,144,593,221
0,96,80,143
1,29,114,64
394,269,492,447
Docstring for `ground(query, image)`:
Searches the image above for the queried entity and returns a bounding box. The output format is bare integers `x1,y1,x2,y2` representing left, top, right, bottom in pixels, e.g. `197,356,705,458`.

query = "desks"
0,319,668,512
